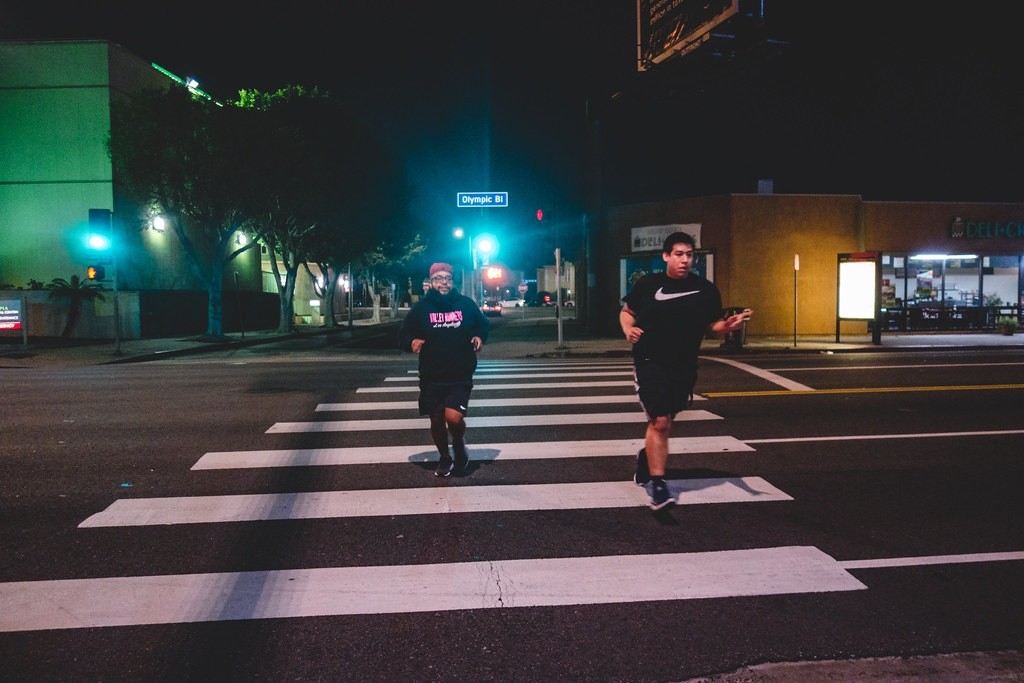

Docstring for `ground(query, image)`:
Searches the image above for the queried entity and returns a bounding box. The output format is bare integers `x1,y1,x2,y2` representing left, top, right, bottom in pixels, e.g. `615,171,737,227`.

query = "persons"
620,231,754,510
398,263,486,476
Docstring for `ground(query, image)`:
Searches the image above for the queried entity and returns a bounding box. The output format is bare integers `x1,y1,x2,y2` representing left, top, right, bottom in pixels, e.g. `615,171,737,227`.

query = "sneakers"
435,456,453,476
453,438,469,470
650,479,676,511
633,447,649,486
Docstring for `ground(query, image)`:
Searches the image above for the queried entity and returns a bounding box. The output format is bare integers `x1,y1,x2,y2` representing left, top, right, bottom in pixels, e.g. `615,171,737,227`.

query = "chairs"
882,302,1018,328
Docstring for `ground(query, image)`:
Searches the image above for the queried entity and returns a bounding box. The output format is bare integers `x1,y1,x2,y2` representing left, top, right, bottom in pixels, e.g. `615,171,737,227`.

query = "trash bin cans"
720,307,752,348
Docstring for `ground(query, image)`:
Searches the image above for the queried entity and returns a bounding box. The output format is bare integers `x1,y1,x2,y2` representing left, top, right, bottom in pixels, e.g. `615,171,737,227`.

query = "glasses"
431,276,453,283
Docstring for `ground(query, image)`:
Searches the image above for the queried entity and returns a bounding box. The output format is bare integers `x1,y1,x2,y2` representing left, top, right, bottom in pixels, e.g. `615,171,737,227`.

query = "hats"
430,263,453,275
